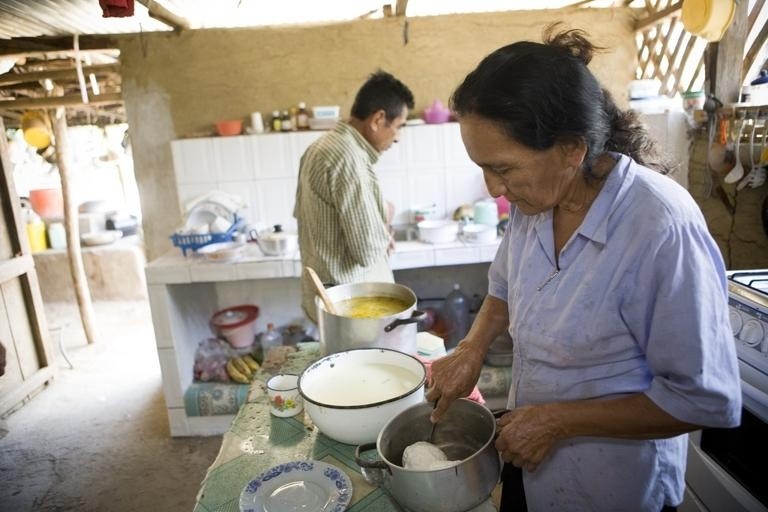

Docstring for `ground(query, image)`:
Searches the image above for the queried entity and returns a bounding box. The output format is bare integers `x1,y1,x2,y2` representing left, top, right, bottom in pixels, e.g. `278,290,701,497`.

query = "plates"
239,458,352,512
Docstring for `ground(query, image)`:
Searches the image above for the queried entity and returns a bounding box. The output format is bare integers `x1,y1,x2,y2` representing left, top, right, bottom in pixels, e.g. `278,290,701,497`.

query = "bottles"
444,284,468,328
471,293,480,311
249,111,264,135
26,209,48,251
49,223,66,250
271,101,309,131
260,322,281,360
424,100,449,124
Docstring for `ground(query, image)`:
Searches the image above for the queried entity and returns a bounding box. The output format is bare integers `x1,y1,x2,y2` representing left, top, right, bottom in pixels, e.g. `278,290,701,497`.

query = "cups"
265,373,304,420
475,202,498,241
682,90,704,115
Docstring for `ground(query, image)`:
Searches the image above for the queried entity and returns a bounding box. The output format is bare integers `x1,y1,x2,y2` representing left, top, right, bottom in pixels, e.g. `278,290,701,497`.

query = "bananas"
227,353,259,383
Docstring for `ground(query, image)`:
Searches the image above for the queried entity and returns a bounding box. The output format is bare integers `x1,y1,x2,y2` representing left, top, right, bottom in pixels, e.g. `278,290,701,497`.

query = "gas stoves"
721,268,768,394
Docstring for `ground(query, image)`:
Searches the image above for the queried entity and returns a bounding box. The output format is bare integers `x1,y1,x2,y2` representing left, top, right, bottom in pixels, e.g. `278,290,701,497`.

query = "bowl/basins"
435,319,458,348
82,229,122,246
485,343,512,366
681,0,736,43
463,222,490,244
296,347,429,445
216,116,243,134
197,242,261,265
387,208,458,245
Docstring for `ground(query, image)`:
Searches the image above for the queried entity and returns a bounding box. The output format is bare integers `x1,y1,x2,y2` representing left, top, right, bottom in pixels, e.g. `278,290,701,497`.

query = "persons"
422,19,743,512
291,68,415,327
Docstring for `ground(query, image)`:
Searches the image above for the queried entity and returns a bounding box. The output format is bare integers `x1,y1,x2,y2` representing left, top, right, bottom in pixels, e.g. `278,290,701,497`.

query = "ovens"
680,350,766,510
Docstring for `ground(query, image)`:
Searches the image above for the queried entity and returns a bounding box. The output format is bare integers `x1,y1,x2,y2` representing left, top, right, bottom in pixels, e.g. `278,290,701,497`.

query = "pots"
249,225,297,255
314,282,427,357
354,392,513,510
